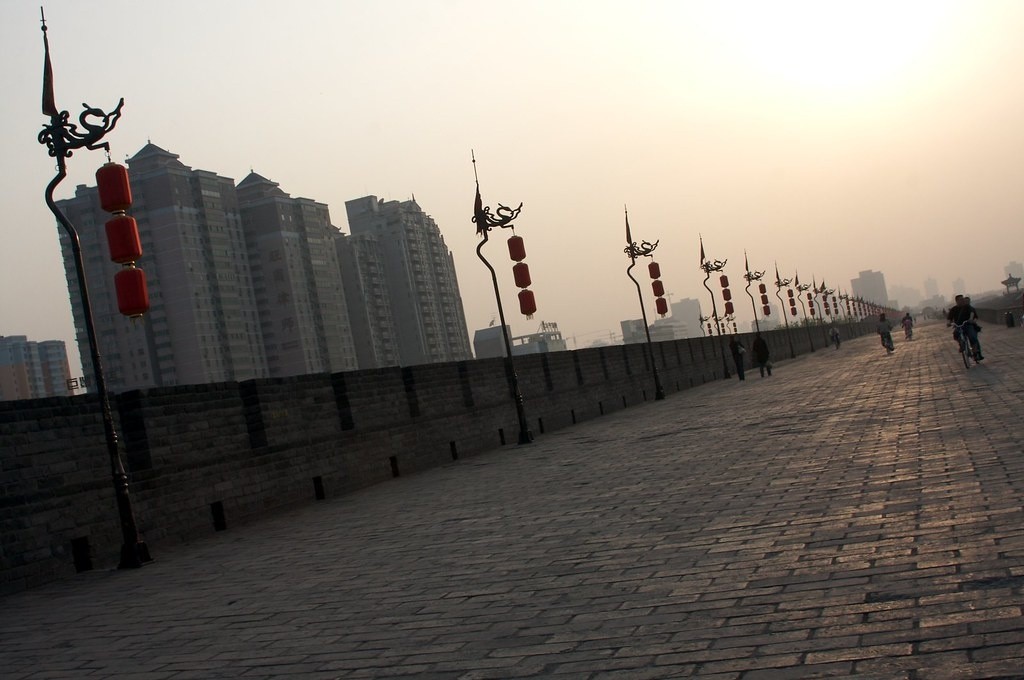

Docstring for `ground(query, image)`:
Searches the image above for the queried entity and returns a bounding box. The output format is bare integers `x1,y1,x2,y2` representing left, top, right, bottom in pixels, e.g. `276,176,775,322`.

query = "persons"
728,335,745,380
753,332,772,378
829,324,841,345
947,296,984,361
902,313,913,339
877,313,894,351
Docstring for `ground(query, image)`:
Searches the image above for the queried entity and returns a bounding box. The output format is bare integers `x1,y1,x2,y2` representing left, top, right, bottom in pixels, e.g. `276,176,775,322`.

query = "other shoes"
973,353,984,361
959,346,965,352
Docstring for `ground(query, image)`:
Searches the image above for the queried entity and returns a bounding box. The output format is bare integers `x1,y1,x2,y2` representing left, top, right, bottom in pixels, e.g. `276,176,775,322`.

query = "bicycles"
948,320,980,368
832,334,839,350
885,340,892,354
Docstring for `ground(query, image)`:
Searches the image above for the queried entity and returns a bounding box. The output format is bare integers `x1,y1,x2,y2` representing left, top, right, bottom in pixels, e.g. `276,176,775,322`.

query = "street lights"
743,248,771,332
773,258,798,330
698,232,734,379
794,269,816,352
623,203,668,399
471,151,536,444
37,7,152,568
812,272,901,347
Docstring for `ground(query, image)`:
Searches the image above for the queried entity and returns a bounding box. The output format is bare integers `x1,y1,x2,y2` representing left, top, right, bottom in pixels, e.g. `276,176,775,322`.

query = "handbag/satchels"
765,357,773,370
736,341,747,354
954,330,958,340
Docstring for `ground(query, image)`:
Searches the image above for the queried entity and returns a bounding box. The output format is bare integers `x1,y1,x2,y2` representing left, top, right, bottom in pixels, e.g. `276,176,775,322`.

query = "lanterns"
807,293,815,315
508,236,537,320
94,161,151,318
648,262,661,279
788,290,797,315
652,281,664,296
846,299,876,316
720,276,734,314
656,298,668,317
759,284,770,317
832,297,839,314
822,295,830,315
